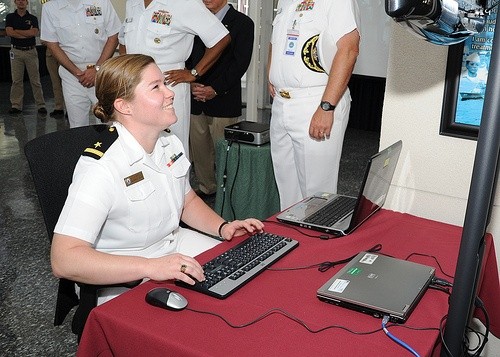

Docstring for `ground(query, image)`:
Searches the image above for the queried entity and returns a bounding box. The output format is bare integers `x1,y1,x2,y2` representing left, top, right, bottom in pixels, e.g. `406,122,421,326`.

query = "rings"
320,132,323,133
181,265,186,272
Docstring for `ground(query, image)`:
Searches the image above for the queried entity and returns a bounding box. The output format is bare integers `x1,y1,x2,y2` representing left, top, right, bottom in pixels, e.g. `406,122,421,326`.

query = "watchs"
191,69,200,79
94,66,99,71
320,101,335,111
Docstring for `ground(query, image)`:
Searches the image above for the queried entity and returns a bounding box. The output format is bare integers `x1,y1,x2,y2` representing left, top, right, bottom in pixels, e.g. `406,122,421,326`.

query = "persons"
6,0,47,114
185,0,254,198
461,46,487,82
41,0,123,129
266,0,362,212
118,0,231,158
51,54,265,307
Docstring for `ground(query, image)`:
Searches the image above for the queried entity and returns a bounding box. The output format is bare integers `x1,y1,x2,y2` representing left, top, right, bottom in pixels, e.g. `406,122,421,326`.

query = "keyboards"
173,232,300,299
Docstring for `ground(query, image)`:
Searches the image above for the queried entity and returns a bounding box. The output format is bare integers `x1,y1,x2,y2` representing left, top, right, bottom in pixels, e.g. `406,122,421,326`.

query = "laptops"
317,252,436,323
278,140,403,237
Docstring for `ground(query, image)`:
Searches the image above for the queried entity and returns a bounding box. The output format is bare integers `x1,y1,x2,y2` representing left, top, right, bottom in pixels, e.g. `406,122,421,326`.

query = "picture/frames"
439,0,498,140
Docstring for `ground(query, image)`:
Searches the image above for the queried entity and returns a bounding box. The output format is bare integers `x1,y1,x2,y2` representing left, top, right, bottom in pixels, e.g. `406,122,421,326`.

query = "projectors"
223,120,271,146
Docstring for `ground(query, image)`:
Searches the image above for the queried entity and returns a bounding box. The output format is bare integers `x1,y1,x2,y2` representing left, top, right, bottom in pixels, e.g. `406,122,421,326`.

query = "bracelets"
215,91,218,95
219,221,231,240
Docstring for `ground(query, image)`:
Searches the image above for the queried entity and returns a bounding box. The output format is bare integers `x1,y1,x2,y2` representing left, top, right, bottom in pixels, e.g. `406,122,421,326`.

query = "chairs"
25,125,110,338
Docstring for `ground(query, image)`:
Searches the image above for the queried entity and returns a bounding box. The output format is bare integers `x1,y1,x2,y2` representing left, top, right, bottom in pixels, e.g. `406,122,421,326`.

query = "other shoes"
39,108,47,114
9,107,20,113
194,189,216,198
50,109,64,115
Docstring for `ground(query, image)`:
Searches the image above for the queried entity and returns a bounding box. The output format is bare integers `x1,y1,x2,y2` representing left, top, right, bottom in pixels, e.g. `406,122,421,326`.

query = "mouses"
146,288,189,311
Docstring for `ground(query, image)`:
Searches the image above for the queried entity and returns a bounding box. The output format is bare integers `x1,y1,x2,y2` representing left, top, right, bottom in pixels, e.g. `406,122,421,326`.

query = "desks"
215,138,280,222
74,205,500,357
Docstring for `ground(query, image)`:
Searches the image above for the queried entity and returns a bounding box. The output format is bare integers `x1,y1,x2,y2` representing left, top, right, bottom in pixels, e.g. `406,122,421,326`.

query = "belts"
13,46,33,50
